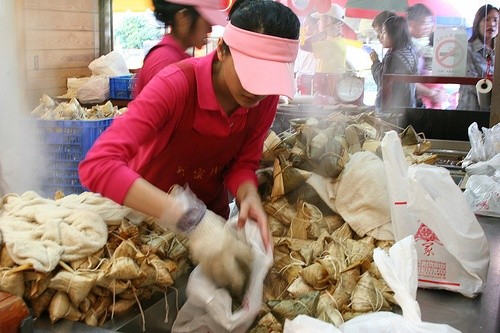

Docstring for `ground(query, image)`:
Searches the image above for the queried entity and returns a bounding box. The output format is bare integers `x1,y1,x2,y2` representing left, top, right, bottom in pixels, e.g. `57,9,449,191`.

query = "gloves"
188,209,253,297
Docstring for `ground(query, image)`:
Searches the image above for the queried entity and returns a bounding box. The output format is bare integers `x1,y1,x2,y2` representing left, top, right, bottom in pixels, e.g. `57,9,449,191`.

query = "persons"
299,4,500,113
131,0,228,100
77,0,302,298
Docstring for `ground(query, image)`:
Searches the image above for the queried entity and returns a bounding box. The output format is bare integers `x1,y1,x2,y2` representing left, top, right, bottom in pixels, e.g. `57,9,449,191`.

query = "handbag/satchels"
170,214,274,333
380,130,490,299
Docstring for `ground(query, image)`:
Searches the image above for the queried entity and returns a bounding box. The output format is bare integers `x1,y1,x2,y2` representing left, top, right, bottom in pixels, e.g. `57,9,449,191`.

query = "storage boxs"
109,74,136,99
17,118,113,199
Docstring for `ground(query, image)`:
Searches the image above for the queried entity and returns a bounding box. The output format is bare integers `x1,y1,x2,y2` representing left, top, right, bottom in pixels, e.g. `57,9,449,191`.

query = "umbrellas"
111,0,459,20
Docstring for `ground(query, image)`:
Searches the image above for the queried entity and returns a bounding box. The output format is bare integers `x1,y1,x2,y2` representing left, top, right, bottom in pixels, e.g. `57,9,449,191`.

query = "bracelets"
175,201,207,234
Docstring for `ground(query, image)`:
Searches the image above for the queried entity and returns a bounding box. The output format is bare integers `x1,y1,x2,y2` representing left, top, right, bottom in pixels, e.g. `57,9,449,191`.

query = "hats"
320,4,346,22
167,0,227,28
223,22,299,102
